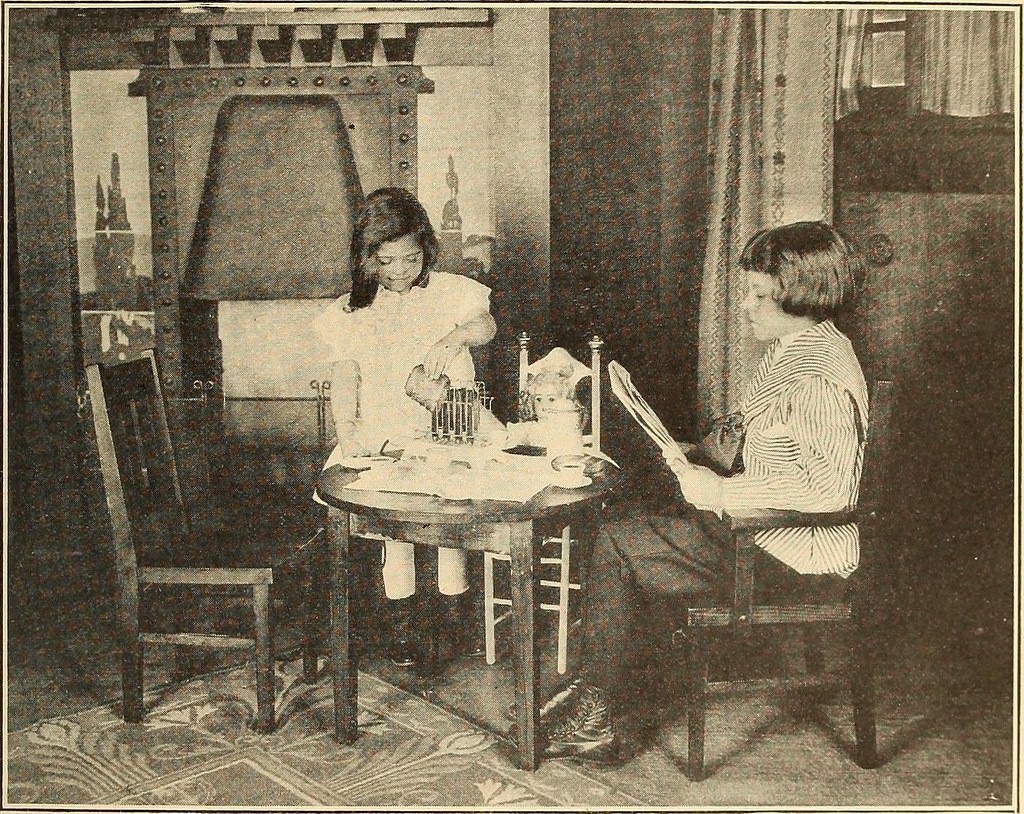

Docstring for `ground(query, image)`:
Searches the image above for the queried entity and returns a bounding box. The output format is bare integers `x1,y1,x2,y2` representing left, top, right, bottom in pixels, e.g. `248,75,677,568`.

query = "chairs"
83,348,330,737
637,380,895,784
478,330,607,675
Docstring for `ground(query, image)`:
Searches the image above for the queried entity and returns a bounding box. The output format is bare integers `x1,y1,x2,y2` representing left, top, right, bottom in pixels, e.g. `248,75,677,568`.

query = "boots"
505,672,622,773
437,587,484,656
385,591,420,666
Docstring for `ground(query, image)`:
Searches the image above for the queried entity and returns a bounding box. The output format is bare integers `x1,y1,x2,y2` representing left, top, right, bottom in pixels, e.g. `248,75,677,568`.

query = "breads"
404,364,478,436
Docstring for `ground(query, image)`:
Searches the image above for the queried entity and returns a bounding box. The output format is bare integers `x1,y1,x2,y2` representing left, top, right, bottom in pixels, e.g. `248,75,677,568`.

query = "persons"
517,373,590,434
319,187,499,666
506,221,869,766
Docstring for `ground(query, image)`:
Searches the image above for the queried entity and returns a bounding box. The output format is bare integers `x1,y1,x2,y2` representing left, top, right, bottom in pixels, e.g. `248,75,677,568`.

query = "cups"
426,447,451,469
370,458,394,472
405,366,450,412
561,462,585,482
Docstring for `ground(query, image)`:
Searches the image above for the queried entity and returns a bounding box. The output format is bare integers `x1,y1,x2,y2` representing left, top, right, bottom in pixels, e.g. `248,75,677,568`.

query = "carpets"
6,639,651,806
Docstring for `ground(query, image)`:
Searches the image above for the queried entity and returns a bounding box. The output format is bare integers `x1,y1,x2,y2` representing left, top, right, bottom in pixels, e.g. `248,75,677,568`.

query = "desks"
316,445,626,773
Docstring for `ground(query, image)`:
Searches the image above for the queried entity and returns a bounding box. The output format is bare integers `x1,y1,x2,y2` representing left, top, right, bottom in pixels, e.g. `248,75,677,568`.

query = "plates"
550,475,592,488
359,470,398,481
